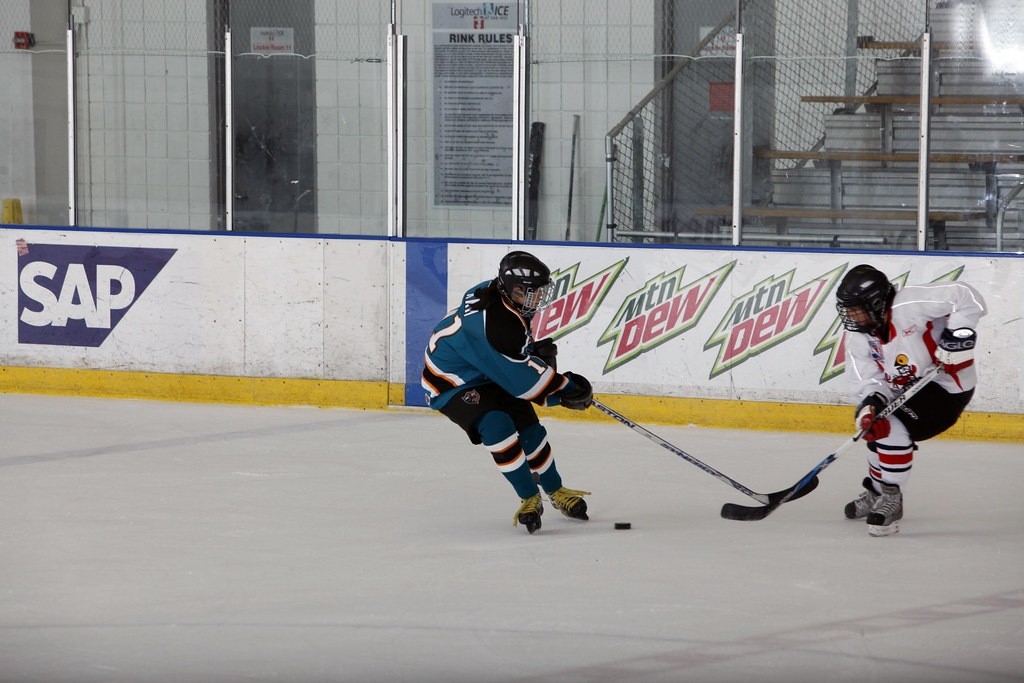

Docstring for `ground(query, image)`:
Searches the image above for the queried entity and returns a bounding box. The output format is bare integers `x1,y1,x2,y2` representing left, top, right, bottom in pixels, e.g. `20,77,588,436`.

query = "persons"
420,251,593,525
836,264,988,525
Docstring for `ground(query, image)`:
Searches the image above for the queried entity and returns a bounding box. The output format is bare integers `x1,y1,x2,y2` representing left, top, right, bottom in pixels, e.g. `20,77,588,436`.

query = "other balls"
614,522,631,530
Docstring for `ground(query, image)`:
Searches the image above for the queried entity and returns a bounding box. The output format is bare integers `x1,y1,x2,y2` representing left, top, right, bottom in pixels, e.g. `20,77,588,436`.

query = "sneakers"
866,481,904,538
512,490,548,533
845,477,881,520
547,487,591,522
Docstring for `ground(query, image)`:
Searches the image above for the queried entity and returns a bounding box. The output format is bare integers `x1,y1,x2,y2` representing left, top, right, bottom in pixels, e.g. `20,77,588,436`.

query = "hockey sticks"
719,362,946,523
591,397,821,508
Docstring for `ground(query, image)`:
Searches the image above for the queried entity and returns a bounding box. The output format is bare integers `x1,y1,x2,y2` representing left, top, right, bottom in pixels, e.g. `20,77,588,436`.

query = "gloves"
529,337,558,374
934,326,977,375
548,372,593,411
855,395,891,441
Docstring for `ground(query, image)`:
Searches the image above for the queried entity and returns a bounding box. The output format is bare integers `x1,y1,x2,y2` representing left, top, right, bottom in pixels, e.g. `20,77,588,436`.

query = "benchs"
686,0,1024,251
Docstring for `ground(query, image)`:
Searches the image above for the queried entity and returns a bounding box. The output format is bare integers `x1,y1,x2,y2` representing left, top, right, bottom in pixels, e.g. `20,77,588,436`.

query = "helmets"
497,251,554,319
835,265,896,337
